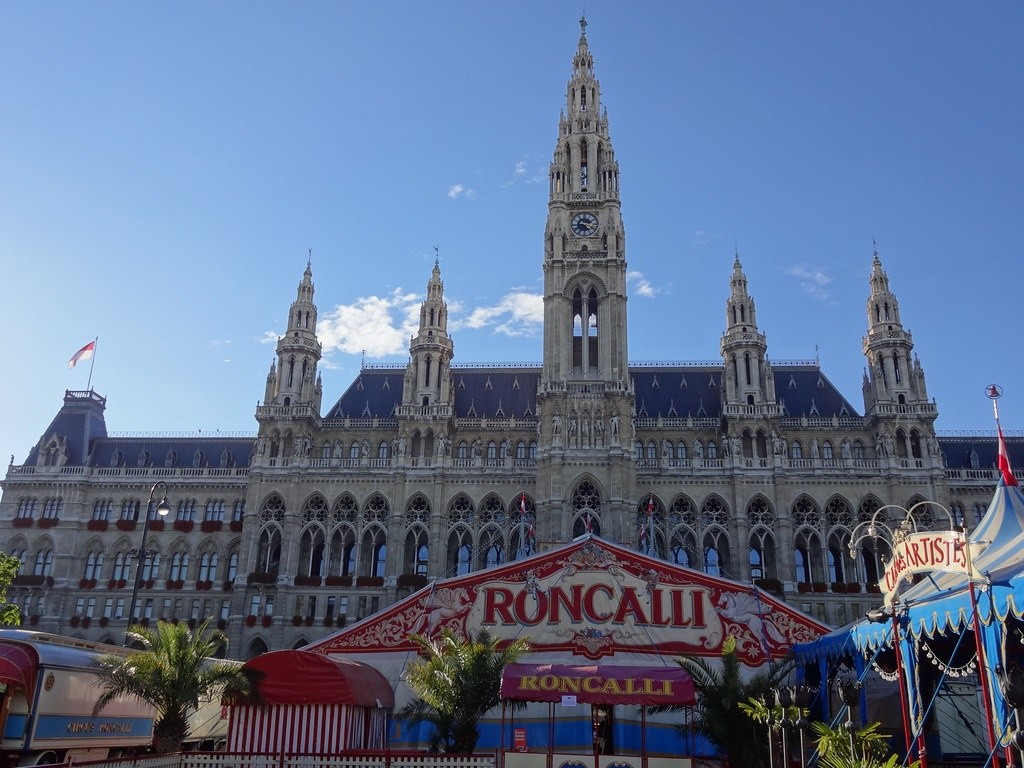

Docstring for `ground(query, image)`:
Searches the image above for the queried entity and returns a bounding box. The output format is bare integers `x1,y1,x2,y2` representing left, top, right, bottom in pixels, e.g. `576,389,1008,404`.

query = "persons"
631,419,636,439
773,435,787,456
886,434,895,456
552,411,562,434
475,434,483,454
294,438,303,457
400,437,406,456
583,415,590,435
842,436,850,458
306,436,311,452
569,414,575,437
595,416,601,436
731,434,739,455
437,435,445,456
505,437,513,456
927,435,940,455
610,412,622,435
391,434,399,457
874,437,884,457
812,438,820,457
257,435,264,456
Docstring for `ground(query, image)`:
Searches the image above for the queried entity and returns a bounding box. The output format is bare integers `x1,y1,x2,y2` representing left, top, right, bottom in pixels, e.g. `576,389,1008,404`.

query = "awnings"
0,640,39,712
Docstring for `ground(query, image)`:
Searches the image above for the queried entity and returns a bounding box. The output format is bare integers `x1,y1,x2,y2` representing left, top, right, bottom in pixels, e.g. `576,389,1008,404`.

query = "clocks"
570,211,600,237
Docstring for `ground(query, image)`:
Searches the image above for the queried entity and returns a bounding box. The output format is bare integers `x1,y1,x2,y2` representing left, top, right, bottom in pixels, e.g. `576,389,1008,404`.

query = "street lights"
121,480,170,647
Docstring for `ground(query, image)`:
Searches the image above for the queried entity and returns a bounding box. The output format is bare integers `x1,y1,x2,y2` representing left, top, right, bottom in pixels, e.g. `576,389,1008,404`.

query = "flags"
521,496,525,515
67,341,94,367
529,522,533,536
648,497,653,512
641,524,645,538
998,425,1019,486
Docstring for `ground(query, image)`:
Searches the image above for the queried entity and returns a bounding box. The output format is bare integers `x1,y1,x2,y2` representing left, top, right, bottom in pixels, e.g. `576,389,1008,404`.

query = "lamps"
865,607,892,624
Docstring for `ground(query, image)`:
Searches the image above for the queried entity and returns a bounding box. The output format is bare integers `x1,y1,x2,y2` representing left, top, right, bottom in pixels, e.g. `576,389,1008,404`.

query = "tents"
789,471,1024,768
296,533,835,750
224,651,394,755
